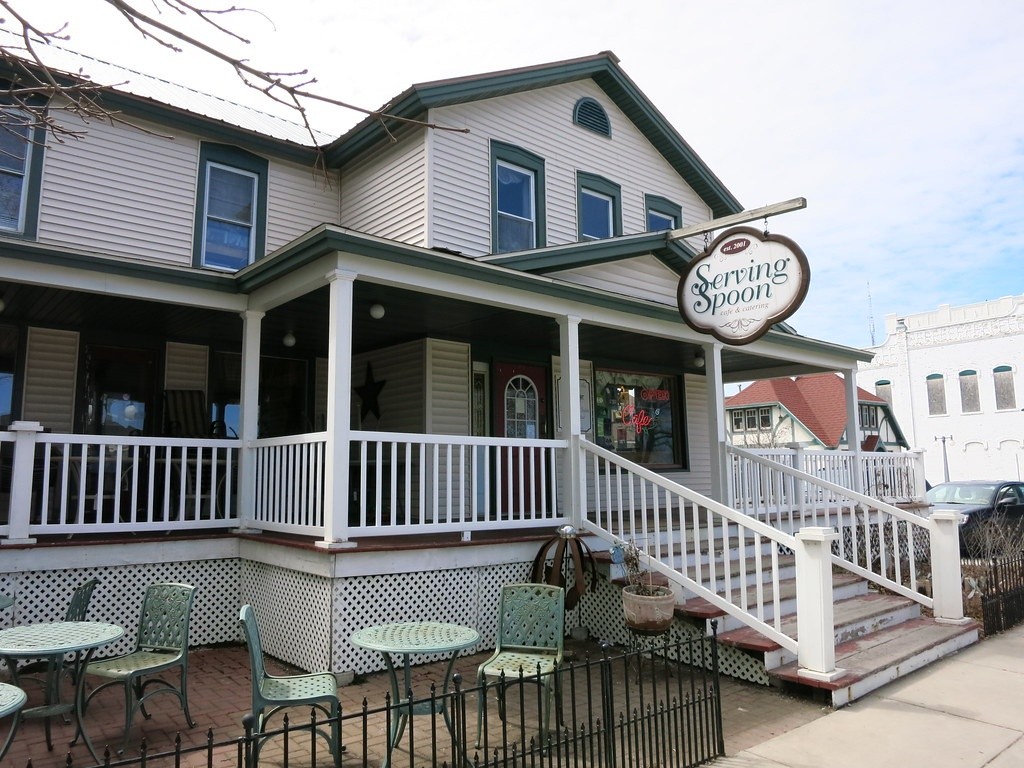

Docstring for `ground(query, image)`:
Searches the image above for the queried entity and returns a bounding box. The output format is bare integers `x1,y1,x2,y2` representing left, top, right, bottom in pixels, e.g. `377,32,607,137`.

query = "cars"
926,479,1024,560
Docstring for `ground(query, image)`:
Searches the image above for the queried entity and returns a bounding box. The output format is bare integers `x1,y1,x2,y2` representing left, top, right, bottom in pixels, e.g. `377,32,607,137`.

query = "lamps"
125,400,138,420
282,328,297,346
369,303,385,320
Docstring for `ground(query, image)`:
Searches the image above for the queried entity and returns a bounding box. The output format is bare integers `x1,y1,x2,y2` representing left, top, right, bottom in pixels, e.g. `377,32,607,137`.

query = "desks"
0,682,28,762
599,450,635,466
350,622,481,768
51,455,239,468
0,594,14,610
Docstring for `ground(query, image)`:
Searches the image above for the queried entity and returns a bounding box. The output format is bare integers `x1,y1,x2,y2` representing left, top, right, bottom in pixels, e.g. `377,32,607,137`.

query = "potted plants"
610,537,675,638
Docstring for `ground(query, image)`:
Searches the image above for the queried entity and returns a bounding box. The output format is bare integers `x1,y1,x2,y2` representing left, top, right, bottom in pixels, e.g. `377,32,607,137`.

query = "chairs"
474,582,565,747
69,583,199,756
0,620,125,766
958,488,971,498
65,579,102,621
165,455,233,534
237,604,337,754
53,447,136,539
